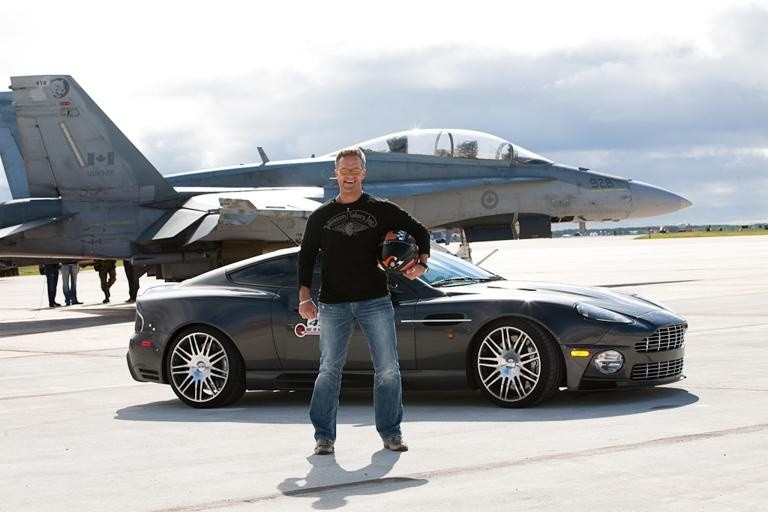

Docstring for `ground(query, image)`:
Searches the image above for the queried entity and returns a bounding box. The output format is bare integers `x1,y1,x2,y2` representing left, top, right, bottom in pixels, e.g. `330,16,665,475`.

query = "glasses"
337,168,364,176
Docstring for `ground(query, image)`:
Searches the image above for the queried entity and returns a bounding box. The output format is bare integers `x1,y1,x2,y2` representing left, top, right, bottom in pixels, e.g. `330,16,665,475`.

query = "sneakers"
50,300,83,307
107,289,110,297
314,438,334,454
125,298,136,302
103,299,109,303
383,438,408,451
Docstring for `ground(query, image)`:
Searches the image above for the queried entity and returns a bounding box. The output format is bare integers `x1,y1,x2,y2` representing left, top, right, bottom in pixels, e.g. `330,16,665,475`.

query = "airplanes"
0,73,692,286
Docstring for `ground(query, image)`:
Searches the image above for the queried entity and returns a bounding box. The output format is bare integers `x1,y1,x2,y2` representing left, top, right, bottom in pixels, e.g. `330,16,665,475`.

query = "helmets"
377,230,421,276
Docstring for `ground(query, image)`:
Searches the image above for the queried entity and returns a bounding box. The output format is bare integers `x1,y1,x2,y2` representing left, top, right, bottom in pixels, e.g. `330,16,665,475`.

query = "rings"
412,267,416,272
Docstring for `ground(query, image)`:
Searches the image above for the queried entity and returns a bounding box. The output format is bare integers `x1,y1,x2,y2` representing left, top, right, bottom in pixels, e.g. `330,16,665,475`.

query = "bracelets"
298,297,314,306
417,259,430,273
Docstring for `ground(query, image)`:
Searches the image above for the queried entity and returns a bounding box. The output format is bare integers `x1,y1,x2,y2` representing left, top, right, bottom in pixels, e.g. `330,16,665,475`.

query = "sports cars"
118,216,693,401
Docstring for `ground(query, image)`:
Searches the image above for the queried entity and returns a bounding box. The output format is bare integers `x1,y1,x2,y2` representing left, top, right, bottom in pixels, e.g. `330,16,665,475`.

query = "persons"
122,259,140,303
92,260,117,303
43,263,62,307
58,262,85,307
294,145,433,453
